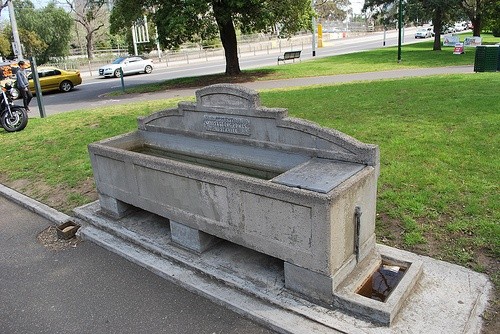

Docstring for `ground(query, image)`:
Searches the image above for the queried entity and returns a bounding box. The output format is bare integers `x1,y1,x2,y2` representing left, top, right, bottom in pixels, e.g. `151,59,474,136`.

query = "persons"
16,61,33,111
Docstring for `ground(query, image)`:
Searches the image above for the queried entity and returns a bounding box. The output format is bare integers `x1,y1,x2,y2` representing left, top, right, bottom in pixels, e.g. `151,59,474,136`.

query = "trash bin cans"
474,45,499,73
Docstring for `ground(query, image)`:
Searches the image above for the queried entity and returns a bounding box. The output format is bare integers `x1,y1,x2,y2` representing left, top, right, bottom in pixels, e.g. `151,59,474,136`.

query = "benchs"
278,51,300,66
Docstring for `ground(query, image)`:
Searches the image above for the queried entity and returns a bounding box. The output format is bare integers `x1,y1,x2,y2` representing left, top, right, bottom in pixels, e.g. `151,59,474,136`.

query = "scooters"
0,81,30,133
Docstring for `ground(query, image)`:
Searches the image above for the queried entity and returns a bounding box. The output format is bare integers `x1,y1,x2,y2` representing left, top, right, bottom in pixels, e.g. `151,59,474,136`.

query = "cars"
6,66,83,101
98,53,156,78
313,26,353,33
415,19,474,39
